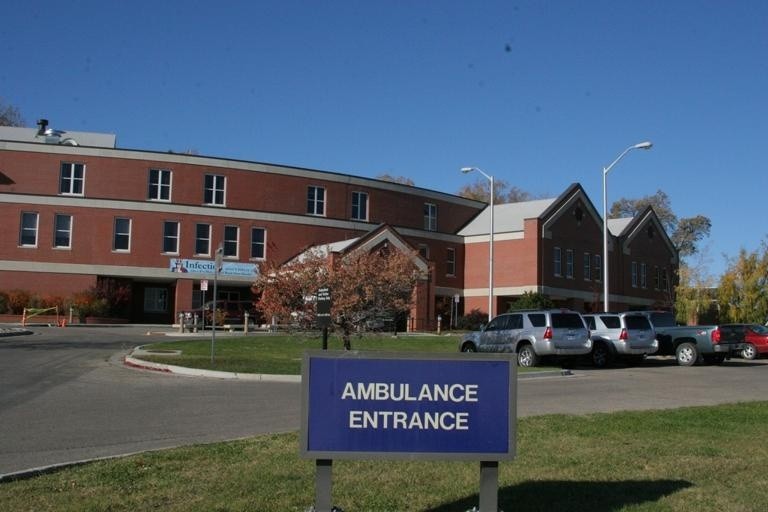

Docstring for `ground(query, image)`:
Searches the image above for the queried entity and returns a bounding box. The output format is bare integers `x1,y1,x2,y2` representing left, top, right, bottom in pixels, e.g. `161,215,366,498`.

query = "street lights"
602,141,653,313
460,166,494,320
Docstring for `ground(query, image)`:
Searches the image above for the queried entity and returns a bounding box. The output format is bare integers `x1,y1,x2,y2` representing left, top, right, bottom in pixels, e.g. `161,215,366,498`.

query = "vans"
570,310,660,369
458,307,594,368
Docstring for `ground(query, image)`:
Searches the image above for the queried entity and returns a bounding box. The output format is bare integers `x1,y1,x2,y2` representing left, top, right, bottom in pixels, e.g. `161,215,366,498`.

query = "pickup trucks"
634,309,747,367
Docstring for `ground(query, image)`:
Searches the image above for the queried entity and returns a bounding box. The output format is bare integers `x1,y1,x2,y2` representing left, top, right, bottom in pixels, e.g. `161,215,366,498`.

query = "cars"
719,321,768,360
